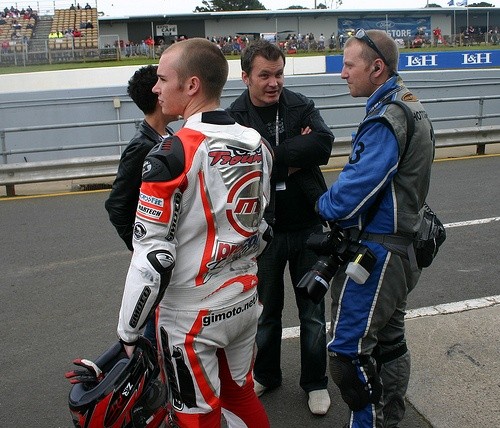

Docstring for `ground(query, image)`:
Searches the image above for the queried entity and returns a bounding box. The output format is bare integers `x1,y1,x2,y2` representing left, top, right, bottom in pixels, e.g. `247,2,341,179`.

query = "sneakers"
309,388,332,415
249,377,267,397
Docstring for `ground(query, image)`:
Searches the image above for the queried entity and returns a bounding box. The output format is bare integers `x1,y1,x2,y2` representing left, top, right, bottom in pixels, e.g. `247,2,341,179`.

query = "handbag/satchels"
414,202,447,268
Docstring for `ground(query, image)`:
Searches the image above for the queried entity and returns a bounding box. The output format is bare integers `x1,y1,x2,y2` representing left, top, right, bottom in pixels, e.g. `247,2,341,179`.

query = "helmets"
65,336,172,428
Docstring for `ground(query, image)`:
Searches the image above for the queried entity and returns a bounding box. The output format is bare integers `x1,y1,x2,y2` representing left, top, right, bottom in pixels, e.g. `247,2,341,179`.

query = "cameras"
295,223,377,305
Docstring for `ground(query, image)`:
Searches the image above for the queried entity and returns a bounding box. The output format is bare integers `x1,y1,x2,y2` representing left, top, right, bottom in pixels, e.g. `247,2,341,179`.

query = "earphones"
373,66,380,72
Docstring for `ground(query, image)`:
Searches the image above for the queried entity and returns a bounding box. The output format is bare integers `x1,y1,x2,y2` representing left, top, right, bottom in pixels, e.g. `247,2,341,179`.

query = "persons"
313,30,435,428
114,38,276,428
104,64,179,253
100,24,500,58
223,39,336,414
0,3,92,54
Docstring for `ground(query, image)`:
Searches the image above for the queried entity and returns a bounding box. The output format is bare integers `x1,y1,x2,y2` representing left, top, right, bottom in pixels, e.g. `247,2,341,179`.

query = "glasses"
352,26,387,61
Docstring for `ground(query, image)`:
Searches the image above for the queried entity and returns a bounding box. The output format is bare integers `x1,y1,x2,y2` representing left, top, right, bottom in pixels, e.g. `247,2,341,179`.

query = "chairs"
0,8,99,53
